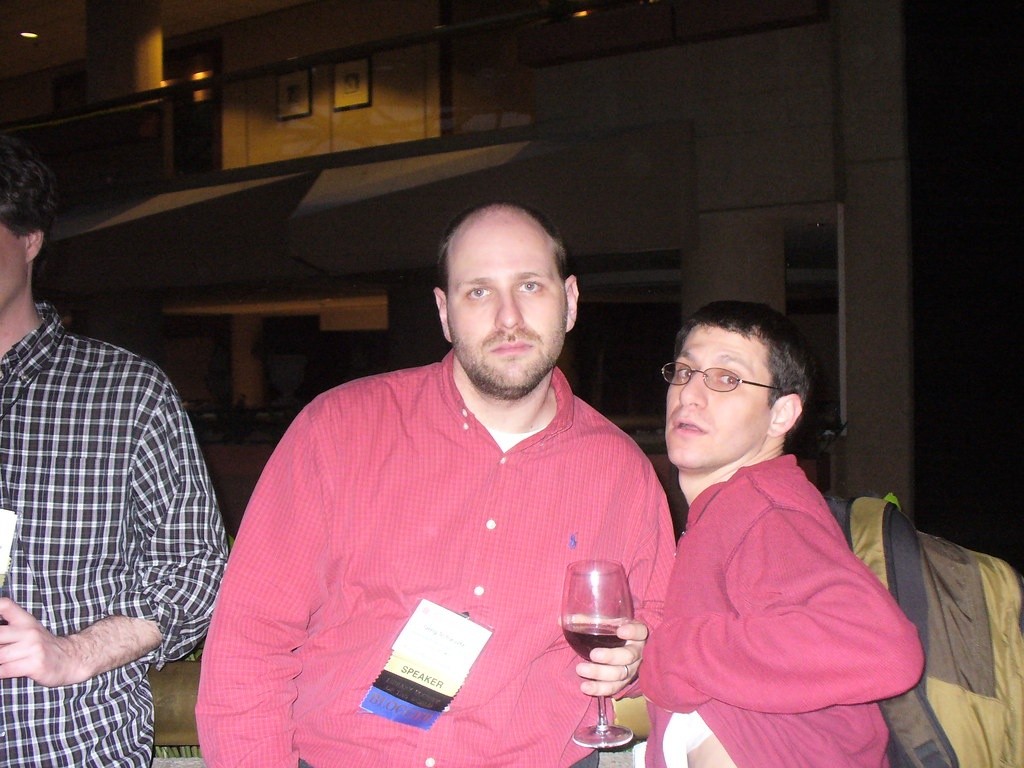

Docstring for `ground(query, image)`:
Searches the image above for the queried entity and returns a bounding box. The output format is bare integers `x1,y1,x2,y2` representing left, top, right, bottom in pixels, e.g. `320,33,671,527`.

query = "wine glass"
562,559,636,750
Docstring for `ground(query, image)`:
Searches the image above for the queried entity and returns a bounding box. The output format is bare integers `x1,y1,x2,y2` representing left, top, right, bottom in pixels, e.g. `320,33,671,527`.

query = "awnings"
32,119,696,291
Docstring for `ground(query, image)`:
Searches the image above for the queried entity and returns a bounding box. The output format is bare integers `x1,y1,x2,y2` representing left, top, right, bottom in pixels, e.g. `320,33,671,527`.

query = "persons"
195,199,677,768
0,135,229,768
576,299,926,768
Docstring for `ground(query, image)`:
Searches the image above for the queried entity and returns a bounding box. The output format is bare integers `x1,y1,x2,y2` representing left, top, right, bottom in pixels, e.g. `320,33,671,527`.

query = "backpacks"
821,494,1024,768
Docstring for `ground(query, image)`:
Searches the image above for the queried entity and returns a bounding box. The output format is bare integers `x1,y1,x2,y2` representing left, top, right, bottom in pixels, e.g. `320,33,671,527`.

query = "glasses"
661,361,781,393
